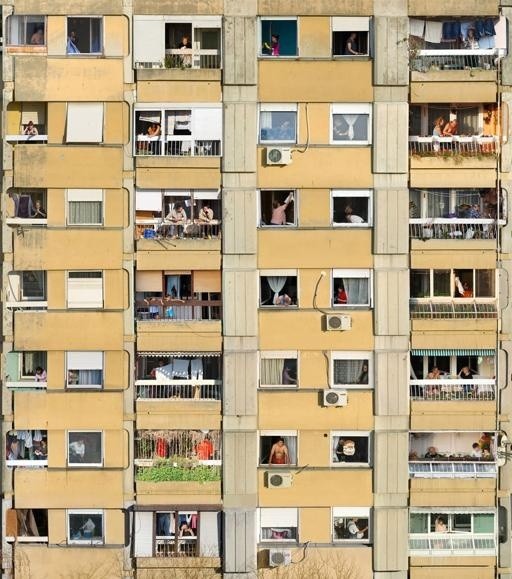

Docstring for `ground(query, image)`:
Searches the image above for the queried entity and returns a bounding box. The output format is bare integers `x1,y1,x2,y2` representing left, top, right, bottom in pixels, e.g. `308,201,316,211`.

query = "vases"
483,448,490,453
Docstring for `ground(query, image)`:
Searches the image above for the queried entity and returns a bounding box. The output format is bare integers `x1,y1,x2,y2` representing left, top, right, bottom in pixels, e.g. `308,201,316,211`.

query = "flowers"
476,435,491,448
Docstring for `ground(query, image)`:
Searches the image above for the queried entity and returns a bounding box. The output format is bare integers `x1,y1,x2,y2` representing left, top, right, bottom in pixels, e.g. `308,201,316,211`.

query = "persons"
150,359,165,377
30,29,44,44
458,29,480,66
282,365,297,384
333,118,349,140
344,214,366,223
264,34,280,56
434,515,447,548
424,446,441,458
423,366,443,397
199,204,213,240
432,117,457,152
179,34,192,68
79,518,95,537
35,366,47,389
347,518,367,538
69,437,85,463
147,123,161,155
275,293,292,309
337,286,347,304
269,193,294,225
143,294,185,319
165,201,187,239
177,522,195,538
458,366,473,397
32,199,46,218
24,120,38,135
35,440,48,460
269,438,291,465
465,204,481,218
344,33,359,55
470,442,483,457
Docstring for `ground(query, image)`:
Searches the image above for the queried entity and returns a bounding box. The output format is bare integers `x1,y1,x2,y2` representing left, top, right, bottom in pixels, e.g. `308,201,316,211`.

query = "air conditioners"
326,313,352,330
269,549,291,566
266,147,291,164
268,472,292,488
323,389,347,407
